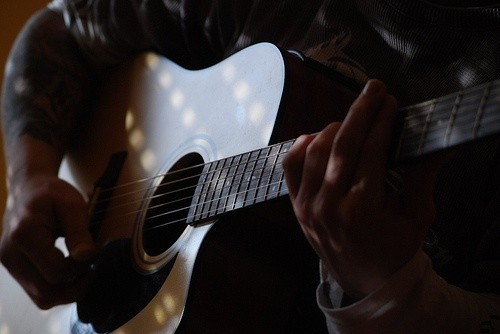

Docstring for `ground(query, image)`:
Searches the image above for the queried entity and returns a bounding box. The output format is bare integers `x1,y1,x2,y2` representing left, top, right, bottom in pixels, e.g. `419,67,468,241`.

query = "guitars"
1,43,499,333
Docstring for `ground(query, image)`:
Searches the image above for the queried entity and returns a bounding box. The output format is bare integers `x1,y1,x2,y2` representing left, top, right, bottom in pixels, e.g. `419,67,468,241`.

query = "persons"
0,0,500,334
